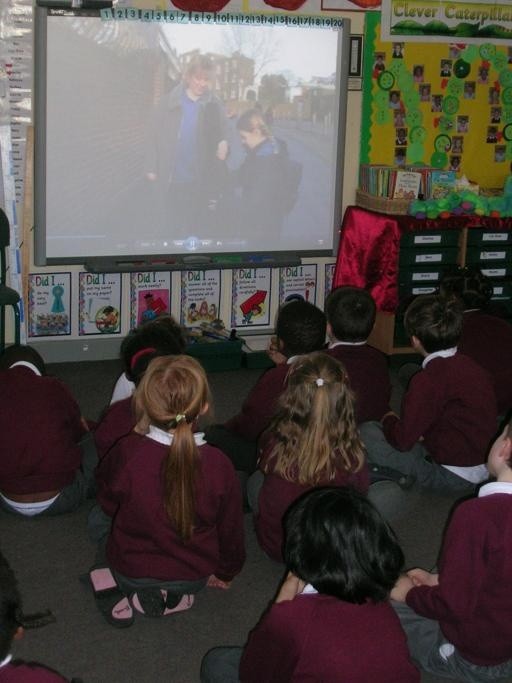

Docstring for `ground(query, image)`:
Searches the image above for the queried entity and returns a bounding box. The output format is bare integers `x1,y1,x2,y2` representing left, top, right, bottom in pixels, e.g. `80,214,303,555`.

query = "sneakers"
130,586,196,617
88,562,134,629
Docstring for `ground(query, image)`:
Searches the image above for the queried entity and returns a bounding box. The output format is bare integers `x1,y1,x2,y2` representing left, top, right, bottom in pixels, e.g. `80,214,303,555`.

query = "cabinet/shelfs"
344,194,512,364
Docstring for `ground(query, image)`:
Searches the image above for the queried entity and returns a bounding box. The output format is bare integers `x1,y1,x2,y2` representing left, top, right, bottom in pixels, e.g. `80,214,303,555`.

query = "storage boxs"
396,230,512,347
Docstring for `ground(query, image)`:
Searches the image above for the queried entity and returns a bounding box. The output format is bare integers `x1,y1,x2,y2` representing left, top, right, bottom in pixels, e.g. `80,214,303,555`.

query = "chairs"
0,207,22,362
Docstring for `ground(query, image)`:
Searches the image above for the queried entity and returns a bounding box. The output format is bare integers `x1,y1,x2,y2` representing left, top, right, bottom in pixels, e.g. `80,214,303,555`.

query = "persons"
391,422,512,683
326,287,392,425
374,41,506,170
216,109,291,250
145,56,228,232
358,271,512,490
204,301,369,562
1,342,101,528
1,555,72,681
89,316,246,627
201,481,422,683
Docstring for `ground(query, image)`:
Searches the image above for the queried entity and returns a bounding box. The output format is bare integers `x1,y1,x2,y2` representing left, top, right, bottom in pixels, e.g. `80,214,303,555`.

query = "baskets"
354,189,421,217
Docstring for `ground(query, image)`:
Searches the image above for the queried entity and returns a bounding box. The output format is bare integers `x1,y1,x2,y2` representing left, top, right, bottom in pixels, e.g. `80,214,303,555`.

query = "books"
358,158,458,202
